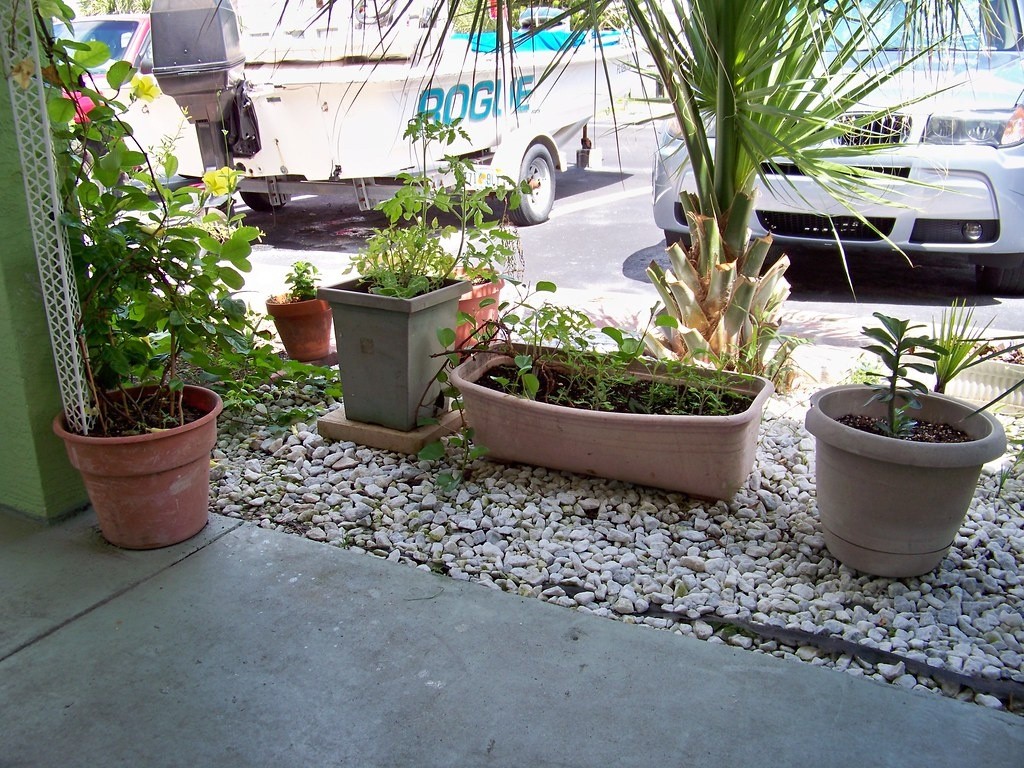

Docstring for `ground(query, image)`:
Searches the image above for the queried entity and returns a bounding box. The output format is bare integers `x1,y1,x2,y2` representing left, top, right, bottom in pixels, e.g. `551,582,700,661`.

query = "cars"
49,9,157,133
654,1,1023,297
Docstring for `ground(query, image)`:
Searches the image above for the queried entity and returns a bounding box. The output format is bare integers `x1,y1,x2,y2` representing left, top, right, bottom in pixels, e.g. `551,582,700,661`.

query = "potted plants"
314,107,536,435
804,312,1010,579
31,0,225,551
265,258,332,361
413,279,775,499
